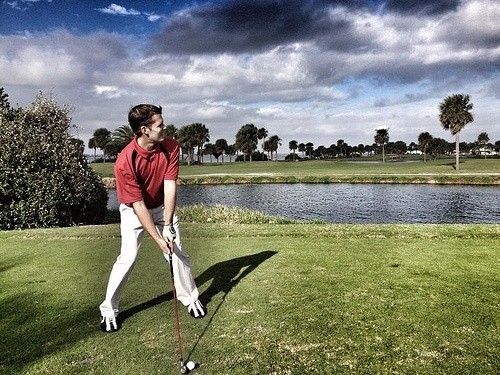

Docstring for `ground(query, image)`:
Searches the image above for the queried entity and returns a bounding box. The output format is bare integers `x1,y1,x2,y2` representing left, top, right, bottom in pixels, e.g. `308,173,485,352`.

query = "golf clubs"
167,243,186,374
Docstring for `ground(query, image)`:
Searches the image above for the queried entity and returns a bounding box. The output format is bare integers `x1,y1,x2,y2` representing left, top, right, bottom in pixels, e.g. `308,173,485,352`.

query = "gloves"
162,223,176,249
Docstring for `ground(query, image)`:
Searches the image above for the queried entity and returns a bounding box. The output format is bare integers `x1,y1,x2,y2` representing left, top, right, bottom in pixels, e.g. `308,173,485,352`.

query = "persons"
97,103,208,333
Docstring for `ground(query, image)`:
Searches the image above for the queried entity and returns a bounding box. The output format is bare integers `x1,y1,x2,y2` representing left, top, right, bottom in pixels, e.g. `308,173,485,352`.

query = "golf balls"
187,361,195,370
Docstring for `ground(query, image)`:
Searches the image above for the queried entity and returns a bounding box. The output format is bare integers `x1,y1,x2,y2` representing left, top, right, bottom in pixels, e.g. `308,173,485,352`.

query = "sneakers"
185,299,205,317
100,315,118,332
